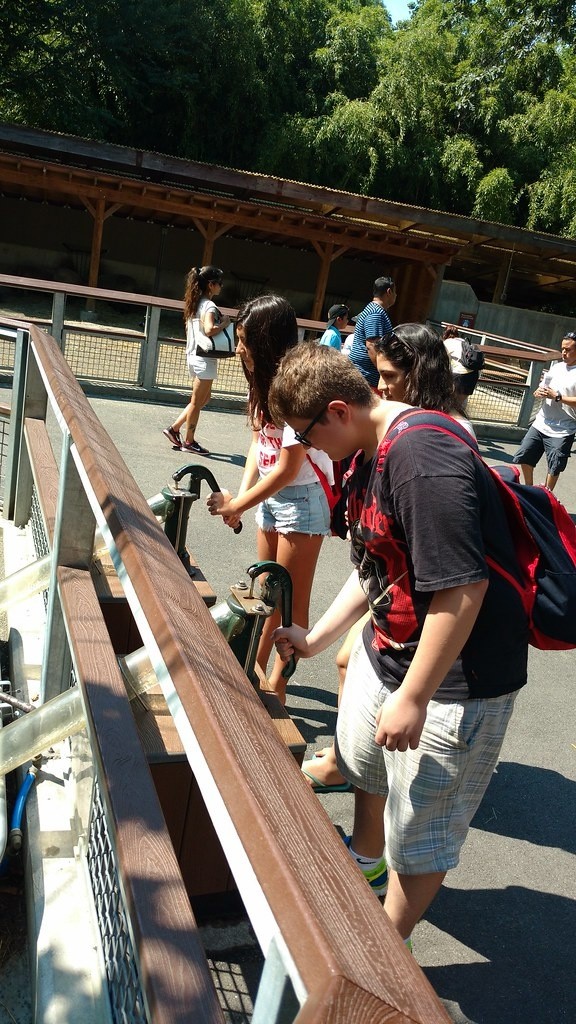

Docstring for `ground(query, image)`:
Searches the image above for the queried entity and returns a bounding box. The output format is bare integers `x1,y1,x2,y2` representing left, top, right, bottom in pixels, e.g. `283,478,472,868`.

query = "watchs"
555,390,561,402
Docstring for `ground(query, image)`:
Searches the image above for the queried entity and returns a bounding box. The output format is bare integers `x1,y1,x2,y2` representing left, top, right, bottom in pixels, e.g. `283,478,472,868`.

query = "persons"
164,266,230,455
269,276,576,953
207,295,336,707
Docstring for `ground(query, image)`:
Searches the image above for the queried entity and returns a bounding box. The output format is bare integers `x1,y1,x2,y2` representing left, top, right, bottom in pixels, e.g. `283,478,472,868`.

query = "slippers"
301,763,351,792
312,747,332,760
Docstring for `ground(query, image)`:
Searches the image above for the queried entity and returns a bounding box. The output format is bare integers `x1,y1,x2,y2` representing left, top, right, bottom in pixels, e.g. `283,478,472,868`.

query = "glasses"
294,399,349,446
379,331,415,353
564,332,576,339
209,279,225,287
388,276,395,289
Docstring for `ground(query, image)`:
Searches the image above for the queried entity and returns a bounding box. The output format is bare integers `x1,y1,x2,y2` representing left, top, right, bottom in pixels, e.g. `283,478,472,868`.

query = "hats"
327,304,350,330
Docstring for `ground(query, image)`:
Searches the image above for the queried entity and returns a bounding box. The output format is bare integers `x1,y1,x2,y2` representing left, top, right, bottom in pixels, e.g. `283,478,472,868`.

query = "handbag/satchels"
192,301,236,358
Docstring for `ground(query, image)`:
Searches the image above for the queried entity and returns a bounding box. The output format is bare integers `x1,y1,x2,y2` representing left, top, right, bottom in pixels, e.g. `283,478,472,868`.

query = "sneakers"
341,838,389,896
180,439,209,455
163,426,182,446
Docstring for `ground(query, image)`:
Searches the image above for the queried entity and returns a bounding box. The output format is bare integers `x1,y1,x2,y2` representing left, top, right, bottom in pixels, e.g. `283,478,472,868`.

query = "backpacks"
346,410,576,650
306,453,355,540
446,337,486,371
